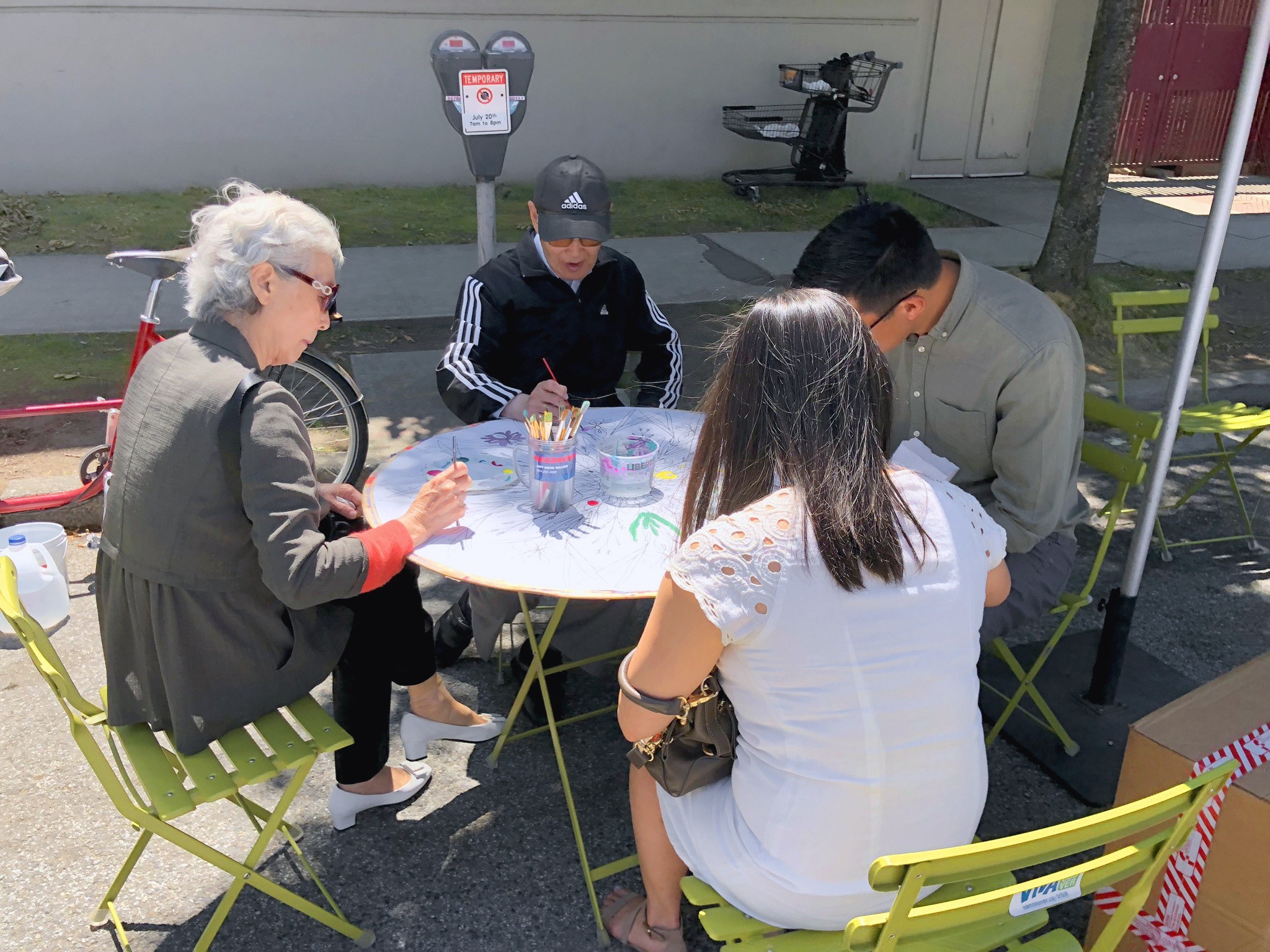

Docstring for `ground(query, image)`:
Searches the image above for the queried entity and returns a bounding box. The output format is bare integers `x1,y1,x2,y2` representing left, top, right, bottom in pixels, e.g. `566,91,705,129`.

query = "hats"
534,154,612,242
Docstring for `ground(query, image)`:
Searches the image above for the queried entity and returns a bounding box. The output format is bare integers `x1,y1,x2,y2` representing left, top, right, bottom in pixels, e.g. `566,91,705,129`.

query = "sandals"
600,890,687,952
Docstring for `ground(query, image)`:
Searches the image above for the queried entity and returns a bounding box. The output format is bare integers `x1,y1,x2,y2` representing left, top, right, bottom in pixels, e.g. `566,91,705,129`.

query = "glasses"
549,237,602,247
266,258,340,314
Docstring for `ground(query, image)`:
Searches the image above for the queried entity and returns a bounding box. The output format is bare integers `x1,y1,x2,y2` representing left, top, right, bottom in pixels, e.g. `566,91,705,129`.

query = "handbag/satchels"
617,648,740,797
820,56,850,89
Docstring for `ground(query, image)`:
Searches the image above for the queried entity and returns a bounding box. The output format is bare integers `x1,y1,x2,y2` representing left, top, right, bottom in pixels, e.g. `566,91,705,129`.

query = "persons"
598,286,1012,952
92,180,509,831
430,155,685,736
787,200,1089,651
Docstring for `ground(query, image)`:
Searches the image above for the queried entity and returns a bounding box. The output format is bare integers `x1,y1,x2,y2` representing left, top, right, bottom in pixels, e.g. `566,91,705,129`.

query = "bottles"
0,534,72,629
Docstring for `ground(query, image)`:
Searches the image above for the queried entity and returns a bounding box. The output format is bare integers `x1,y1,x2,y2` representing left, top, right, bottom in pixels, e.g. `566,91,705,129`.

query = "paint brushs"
452,435,459,526
542,357,584,431
521,400,591,511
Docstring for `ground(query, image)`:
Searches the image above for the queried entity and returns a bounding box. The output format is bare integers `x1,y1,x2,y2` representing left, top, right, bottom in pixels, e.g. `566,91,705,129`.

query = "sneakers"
433,586,474,668
511,634,567,725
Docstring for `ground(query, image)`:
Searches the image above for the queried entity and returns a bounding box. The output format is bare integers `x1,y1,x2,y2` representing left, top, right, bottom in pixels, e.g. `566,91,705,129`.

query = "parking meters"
433,27,537,268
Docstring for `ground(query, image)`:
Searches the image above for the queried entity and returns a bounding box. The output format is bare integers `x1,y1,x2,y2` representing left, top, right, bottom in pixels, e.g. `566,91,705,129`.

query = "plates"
426,454,521,491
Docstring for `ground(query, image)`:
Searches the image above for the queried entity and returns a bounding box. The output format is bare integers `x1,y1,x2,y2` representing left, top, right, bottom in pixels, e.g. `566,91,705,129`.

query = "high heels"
400,706,507,762
327,762,432,831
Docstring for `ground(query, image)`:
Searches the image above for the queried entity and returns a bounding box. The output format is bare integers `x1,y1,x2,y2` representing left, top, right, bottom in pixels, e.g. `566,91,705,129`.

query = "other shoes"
330,312,343,320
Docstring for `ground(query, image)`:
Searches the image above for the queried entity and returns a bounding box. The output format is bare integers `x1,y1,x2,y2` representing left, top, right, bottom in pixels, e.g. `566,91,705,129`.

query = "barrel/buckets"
1,521,68,633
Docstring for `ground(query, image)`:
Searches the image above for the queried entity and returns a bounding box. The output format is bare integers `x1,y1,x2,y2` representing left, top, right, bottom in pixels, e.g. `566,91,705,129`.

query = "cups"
596,434,661,498
513,426,579,513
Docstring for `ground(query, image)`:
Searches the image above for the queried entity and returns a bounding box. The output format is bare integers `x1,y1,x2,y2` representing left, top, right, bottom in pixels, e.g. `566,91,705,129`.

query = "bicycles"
0,244,373,515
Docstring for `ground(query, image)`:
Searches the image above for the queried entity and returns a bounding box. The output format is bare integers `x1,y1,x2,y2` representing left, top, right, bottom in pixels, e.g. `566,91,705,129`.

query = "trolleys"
721,50,903,205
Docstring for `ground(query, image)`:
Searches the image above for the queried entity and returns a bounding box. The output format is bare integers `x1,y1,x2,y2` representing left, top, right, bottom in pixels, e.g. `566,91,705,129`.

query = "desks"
365,397,779,948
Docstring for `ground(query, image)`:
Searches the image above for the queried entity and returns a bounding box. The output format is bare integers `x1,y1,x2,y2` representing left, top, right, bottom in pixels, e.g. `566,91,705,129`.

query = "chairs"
662,757,1244,952
979,386,1165,760
1110,278,1270,578
0,552,361,952
